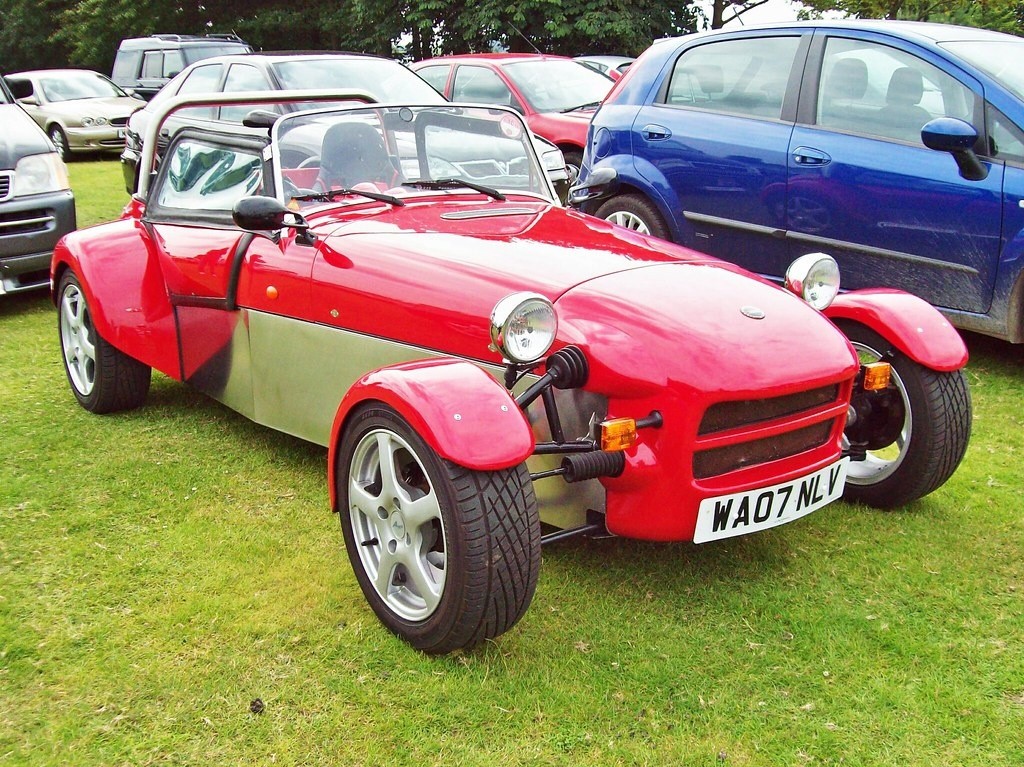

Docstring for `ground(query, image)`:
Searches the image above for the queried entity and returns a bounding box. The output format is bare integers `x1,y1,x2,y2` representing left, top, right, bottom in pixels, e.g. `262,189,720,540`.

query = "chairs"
684,64,748,114
460,69,508,106
311,122,407,194
885,66,932,131
748,78,789,118
825,59,874,123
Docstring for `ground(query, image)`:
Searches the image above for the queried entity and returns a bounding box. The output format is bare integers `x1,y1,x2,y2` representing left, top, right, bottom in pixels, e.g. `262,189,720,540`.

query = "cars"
0,75,84,306
48,83,974,659
3,59,157,159
119,47,571,218
567,18,1024,355
407,41,637,202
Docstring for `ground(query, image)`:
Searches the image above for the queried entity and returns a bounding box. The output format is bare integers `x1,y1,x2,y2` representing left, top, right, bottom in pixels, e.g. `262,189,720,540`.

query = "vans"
109,29,263,98
